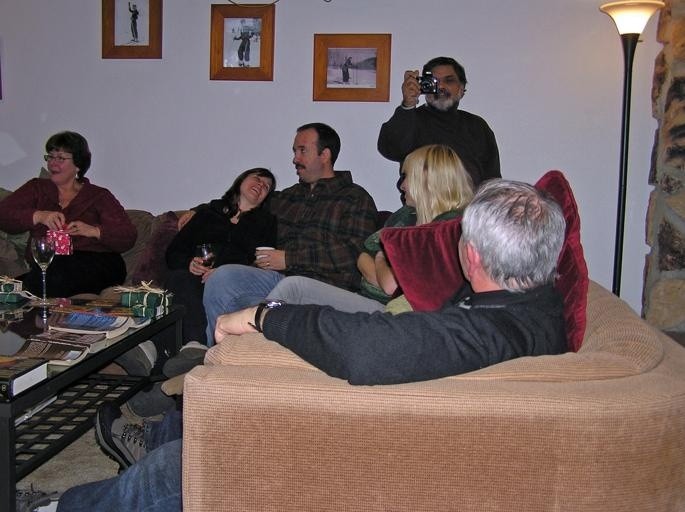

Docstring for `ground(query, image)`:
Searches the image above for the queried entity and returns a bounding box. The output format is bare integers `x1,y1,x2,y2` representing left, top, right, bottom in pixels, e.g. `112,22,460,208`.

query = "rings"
193,262,196,267
72,227,80,232
267,261,270,266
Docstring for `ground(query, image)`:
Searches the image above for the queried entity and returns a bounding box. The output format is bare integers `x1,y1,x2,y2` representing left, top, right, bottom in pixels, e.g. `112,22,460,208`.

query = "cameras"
416,71,439,94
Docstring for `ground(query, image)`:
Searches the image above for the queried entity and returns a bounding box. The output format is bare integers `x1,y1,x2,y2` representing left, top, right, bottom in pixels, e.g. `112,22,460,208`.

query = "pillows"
379,169,589,352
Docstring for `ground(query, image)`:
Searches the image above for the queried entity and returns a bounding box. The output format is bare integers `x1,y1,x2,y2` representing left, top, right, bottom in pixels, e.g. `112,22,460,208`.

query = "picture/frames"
102,0,163,60
210,3,277,81
313,32,392,102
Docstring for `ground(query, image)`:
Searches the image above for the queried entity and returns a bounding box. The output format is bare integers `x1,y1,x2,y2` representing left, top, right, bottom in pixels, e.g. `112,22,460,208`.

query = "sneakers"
89,403,151,474
118,383,173,420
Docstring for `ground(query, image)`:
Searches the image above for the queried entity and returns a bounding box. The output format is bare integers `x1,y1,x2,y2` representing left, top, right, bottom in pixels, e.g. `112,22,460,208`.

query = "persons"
234,19,254,67
341,56,353,82
128,2,140,43
0,130,138,301
120,123,379,426
163,166,276,360
263,144,475,314
375,56,502,204
17,181,569,512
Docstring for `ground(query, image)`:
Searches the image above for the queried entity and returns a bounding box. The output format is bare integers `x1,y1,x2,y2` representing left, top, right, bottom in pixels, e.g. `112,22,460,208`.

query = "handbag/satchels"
46,225,75,256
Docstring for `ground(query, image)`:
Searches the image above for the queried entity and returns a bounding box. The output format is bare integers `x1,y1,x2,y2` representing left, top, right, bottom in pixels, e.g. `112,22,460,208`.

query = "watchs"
247,298,282,332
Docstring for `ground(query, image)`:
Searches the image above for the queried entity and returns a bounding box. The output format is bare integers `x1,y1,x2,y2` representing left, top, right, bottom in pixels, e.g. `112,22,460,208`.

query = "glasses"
42,153,74,164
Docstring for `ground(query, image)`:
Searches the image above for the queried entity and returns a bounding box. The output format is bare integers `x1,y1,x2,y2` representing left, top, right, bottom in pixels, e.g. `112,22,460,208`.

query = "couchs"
1,205,685,512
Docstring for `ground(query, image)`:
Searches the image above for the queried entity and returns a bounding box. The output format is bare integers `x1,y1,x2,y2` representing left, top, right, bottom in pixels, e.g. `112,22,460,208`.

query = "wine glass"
30,236,59,308
194,242,216,283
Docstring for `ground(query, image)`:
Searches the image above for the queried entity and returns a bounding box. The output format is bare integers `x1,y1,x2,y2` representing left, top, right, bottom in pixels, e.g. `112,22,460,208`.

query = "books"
0,354,50,398
14,394,58,428
16,329,108,365
47,312,151,340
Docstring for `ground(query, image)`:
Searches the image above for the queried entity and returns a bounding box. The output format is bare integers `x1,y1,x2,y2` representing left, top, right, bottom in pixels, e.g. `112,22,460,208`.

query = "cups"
253,245,276,270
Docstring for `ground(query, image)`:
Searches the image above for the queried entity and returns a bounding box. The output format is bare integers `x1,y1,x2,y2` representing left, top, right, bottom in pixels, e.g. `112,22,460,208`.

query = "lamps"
599,0,667,299
